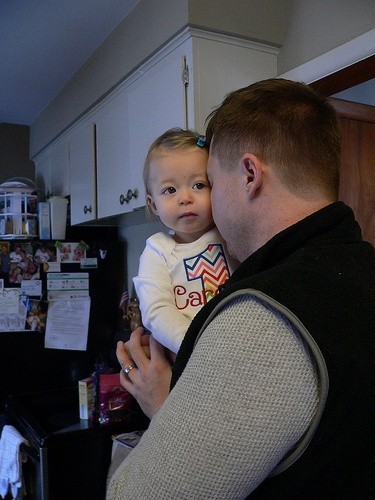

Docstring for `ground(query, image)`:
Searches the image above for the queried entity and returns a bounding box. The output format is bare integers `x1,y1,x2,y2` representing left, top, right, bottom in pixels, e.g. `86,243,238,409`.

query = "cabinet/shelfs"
33,27,280,225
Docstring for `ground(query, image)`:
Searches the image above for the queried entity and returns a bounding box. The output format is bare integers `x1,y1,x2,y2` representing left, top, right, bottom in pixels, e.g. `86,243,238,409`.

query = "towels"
0,425,30,500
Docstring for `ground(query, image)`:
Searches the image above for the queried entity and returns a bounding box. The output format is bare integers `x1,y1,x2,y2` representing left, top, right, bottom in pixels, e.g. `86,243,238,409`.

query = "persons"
132,126,240,363
107,77,375,500
0,243,86,283
26,300,47,331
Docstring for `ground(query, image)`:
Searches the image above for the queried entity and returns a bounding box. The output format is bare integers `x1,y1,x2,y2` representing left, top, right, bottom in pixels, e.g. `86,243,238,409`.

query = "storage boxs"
48,195,69,239
79,377,93,419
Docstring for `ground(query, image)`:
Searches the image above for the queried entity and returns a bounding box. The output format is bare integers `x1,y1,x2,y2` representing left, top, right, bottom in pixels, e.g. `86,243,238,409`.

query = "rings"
124,365,136,374
